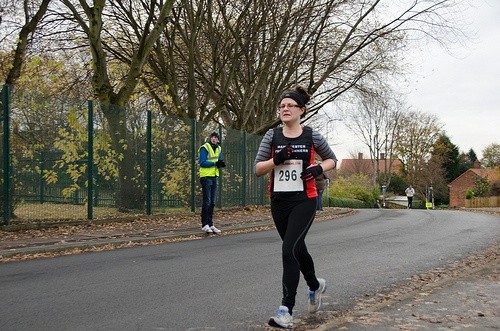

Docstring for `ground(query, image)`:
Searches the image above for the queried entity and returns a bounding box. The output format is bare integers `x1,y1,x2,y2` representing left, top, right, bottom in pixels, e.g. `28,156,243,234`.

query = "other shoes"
201,225,213,233
210,225,221,233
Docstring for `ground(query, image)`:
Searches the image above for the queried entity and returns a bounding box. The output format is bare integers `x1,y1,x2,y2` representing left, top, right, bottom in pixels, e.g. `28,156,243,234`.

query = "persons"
315,172,327,211
405,184,414,208
253,85,338,330
199,131,226,232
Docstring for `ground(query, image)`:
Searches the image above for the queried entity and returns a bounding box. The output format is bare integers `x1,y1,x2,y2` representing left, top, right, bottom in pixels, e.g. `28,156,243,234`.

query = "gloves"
274,142,294,165
216,160,226,169
300,164,323,182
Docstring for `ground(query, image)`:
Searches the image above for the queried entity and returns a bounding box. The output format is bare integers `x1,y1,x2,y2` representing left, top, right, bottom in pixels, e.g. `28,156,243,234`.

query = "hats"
211,131,219,140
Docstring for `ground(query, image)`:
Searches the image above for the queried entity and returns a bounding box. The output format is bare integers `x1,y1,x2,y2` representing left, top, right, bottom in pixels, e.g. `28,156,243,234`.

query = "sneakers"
308,278,327,313
268,306,293,329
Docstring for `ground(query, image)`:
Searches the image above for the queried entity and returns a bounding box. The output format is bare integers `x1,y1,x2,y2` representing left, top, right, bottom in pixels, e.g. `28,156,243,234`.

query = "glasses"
279,103,302,110
